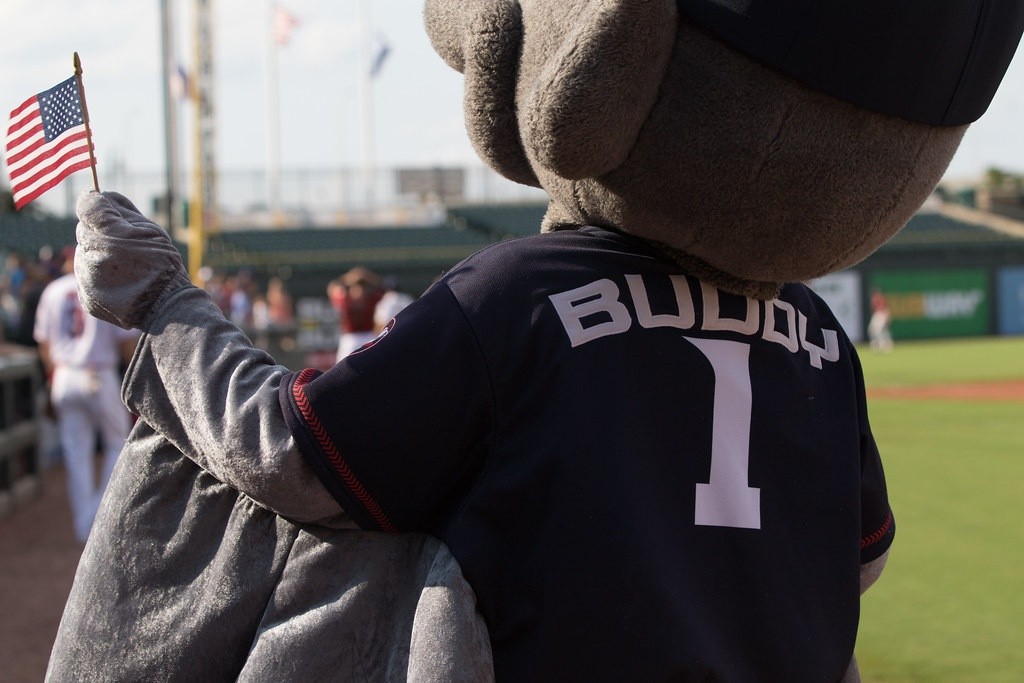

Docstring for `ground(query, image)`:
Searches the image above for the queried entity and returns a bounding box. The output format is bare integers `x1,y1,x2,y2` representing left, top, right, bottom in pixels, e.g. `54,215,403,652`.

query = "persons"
34,245,147,541
2,240,305,370
373,278,414,329
326,265,388,364
868,285,894,349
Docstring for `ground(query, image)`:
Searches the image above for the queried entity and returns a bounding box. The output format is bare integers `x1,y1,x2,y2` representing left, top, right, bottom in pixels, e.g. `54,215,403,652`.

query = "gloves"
71,191,185,331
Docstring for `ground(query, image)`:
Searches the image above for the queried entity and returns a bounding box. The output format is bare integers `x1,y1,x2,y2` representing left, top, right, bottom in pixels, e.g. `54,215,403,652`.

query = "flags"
2,72,98,211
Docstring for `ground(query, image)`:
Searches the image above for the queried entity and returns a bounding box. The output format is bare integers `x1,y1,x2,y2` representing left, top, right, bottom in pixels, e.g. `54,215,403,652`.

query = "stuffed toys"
71,1,1022,682
